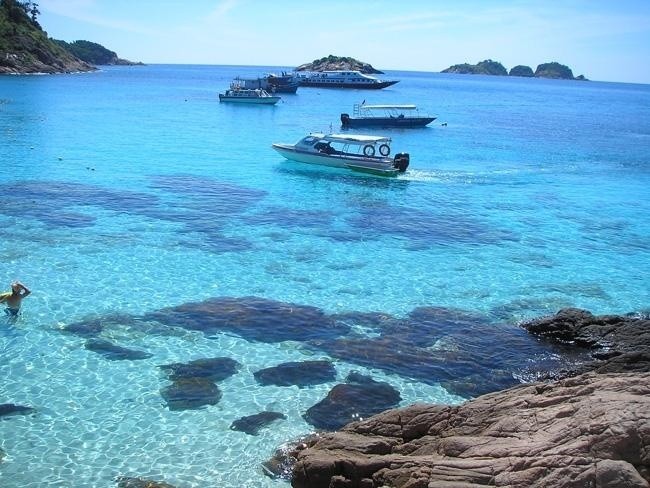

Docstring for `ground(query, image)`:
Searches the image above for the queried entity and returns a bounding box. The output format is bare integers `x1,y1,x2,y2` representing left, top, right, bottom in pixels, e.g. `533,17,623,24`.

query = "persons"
0,279,31,316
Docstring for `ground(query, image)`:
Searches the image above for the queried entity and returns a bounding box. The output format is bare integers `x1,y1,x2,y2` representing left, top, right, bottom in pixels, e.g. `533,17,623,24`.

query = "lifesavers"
379,144,390,156
363,145,375,158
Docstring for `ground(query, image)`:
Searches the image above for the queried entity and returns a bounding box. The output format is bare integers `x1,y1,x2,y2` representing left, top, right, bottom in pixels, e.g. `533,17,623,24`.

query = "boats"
271,132,410,178
264,69,400,89
218,86,281,104
229,75,299,94
340,104,438,128
